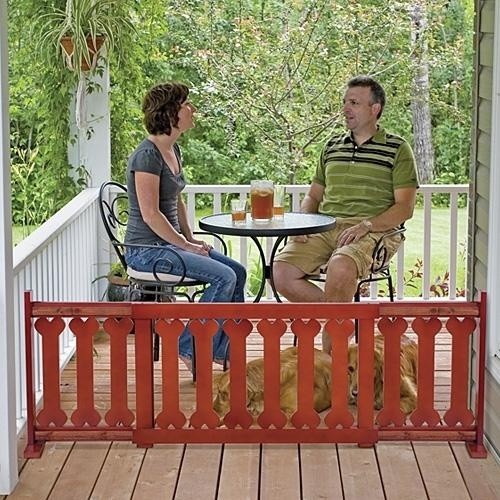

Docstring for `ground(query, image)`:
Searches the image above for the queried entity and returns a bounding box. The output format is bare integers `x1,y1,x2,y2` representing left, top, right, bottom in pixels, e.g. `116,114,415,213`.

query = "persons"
269,74,421,353
121,82,248,376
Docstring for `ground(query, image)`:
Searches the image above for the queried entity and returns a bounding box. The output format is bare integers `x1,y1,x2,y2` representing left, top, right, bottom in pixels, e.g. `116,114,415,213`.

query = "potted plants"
90,229,162,334
28,0,146,82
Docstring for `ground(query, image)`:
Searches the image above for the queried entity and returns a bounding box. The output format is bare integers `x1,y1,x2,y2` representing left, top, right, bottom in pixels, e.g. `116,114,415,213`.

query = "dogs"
348,334,418,425
213,346,332,427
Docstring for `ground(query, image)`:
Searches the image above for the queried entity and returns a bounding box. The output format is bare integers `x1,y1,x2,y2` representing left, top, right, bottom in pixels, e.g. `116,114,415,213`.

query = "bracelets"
363,219,372,231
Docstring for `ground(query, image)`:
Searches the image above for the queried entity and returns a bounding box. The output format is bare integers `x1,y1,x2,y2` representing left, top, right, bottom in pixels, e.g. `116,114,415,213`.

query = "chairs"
99,181,227,381
293,220,406,348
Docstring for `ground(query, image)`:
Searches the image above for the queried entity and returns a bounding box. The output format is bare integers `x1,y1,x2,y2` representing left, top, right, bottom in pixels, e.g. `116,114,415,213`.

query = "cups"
273,186,284,218
230,199,247,223
250,179,274,222
